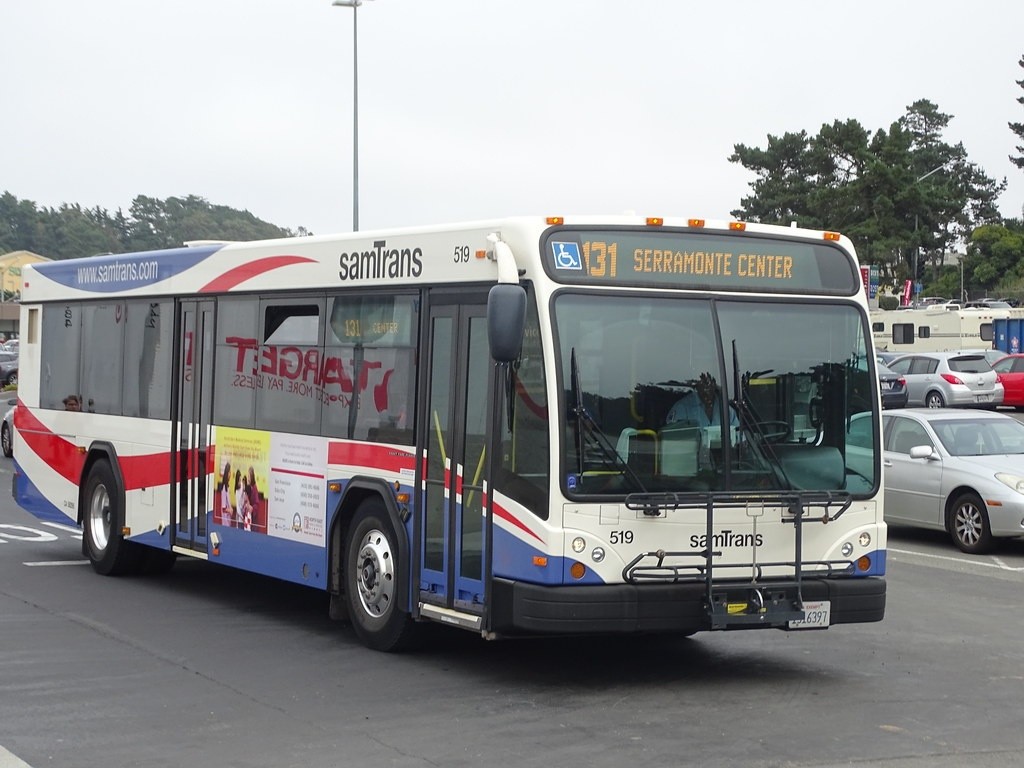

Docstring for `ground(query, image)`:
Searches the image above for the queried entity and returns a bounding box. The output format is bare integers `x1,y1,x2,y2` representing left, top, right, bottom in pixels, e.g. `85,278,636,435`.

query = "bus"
11,216,891,656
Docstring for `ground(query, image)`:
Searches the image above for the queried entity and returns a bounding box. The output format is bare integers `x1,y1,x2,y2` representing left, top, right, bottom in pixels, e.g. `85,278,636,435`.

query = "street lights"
331,0,363,233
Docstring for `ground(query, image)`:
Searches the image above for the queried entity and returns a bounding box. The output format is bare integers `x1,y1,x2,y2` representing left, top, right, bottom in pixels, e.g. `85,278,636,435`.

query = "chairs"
897,432,920,452
953,426,979,455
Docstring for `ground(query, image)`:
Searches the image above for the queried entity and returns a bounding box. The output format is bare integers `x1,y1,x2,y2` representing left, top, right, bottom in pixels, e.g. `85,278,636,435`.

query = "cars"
0,332,19,387
790,295,1023,413
1,396,17,458
794,406,1023,555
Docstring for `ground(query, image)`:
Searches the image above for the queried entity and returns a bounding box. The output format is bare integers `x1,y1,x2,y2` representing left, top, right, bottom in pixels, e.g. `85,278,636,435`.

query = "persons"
666,363,747,443
62,395,81,412
221,462,261,532
